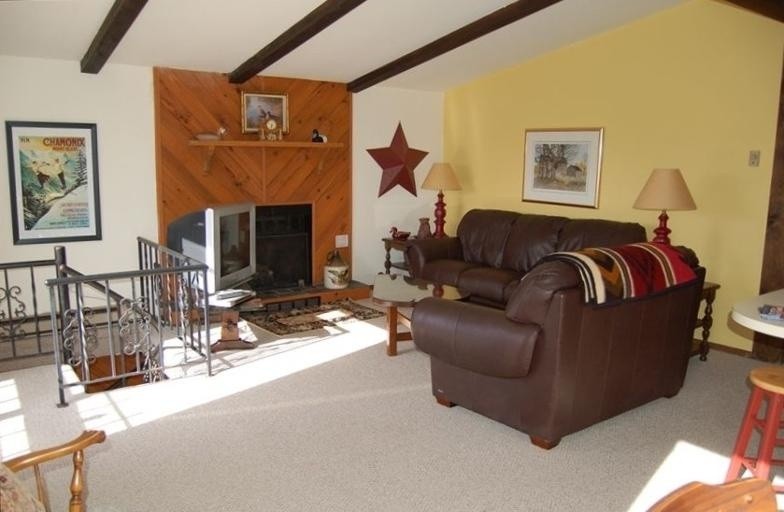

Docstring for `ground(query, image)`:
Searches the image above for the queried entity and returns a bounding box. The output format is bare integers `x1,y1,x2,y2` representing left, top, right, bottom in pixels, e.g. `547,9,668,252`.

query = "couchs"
412,246,706,449
404,209,646,309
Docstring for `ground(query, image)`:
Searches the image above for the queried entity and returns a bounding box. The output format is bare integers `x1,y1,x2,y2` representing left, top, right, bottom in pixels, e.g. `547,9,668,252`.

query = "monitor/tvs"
167,203,256,295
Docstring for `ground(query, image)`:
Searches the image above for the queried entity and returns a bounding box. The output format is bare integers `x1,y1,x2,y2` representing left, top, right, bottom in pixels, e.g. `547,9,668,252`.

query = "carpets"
239,297,386,335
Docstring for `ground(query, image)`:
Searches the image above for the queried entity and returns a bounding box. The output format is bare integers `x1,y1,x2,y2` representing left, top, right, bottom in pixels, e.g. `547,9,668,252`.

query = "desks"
731,289,784,339
187,283,268,353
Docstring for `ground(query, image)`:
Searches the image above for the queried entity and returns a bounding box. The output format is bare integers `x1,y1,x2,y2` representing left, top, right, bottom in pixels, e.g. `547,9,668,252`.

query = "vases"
417,218,431,240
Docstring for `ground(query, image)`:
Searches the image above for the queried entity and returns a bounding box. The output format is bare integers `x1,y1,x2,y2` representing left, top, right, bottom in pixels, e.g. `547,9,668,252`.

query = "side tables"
382,236,417,274
688,282,720,361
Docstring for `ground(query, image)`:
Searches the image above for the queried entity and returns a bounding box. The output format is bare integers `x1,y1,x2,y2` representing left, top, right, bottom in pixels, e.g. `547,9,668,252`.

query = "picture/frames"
6,121,102,244
522,128,603,208
241,90,290,135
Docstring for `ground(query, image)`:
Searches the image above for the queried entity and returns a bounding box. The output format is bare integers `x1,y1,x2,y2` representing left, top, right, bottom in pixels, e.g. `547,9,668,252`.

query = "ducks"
389,227,411,239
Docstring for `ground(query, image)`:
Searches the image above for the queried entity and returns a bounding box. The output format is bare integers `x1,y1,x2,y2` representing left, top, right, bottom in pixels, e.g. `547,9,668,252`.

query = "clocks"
258,117,282,141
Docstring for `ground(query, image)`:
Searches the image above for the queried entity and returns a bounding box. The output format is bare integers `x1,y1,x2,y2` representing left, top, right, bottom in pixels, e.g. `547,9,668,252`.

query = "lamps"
421,164,462,240
633,169,697,244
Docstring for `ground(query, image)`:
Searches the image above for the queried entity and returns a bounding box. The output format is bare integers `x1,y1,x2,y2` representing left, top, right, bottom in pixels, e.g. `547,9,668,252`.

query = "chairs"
645,477,778,512
0,431,109,512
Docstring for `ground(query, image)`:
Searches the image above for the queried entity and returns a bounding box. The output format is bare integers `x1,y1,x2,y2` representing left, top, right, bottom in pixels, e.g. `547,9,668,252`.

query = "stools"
724,366,784,492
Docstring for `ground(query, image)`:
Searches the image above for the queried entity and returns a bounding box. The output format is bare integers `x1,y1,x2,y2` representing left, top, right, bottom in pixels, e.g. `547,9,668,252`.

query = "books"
203,289,256,308
